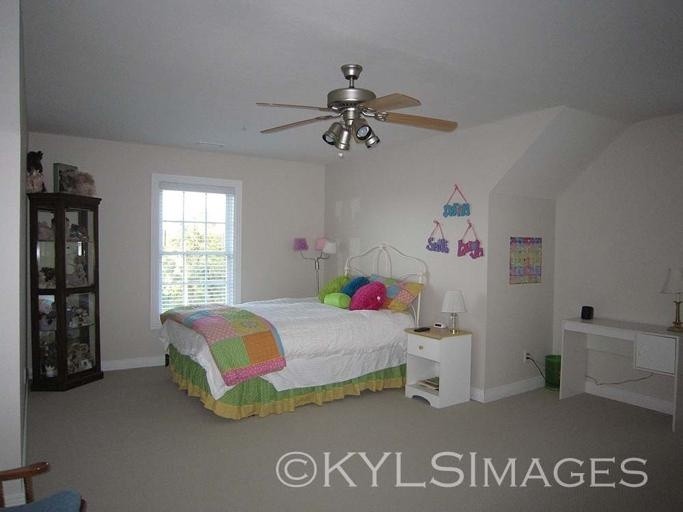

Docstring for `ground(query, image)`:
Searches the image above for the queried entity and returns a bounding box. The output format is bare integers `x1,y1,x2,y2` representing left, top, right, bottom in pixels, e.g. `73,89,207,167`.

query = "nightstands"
404,325,473,408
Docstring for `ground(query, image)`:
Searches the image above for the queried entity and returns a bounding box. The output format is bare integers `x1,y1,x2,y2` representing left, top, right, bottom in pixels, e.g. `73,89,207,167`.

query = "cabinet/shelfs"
559,317,683,432
25,191,102,391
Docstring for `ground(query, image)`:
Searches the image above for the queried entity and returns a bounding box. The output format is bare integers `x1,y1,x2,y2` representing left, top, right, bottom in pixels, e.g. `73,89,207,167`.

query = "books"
417,376,439,390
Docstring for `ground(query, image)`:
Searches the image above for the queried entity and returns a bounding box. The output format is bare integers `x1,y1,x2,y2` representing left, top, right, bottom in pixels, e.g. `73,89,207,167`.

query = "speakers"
581,306,593,319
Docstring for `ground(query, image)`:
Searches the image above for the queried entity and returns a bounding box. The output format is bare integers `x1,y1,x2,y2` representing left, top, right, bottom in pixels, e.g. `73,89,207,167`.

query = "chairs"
0,460,82,512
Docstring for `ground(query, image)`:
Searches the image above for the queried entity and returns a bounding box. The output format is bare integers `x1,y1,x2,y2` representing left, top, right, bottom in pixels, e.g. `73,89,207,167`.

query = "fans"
255,64,458,134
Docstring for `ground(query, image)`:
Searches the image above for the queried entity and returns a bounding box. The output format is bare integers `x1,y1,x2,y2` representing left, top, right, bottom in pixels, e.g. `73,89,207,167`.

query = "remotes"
414,327,430,332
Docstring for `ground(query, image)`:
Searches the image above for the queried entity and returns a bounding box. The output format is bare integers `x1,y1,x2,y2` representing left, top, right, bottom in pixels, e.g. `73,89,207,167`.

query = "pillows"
319,273,426,310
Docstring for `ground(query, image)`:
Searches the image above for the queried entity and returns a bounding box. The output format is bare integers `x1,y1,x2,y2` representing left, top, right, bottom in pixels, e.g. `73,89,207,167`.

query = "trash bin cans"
544,354,560,390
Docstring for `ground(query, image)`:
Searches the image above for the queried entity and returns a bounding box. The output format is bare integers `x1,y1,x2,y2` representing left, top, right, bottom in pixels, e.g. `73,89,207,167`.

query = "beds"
160,241,427,420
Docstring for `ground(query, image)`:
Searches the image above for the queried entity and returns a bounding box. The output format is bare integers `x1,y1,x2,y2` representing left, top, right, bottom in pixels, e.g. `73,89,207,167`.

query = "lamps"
322,110,380,151
441,290,467,335
659,268,683,331
294,235,336,294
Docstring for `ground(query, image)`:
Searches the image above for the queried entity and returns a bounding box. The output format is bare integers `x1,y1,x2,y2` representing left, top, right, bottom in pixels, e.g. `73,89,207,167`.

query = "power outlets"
524,351,531,361
